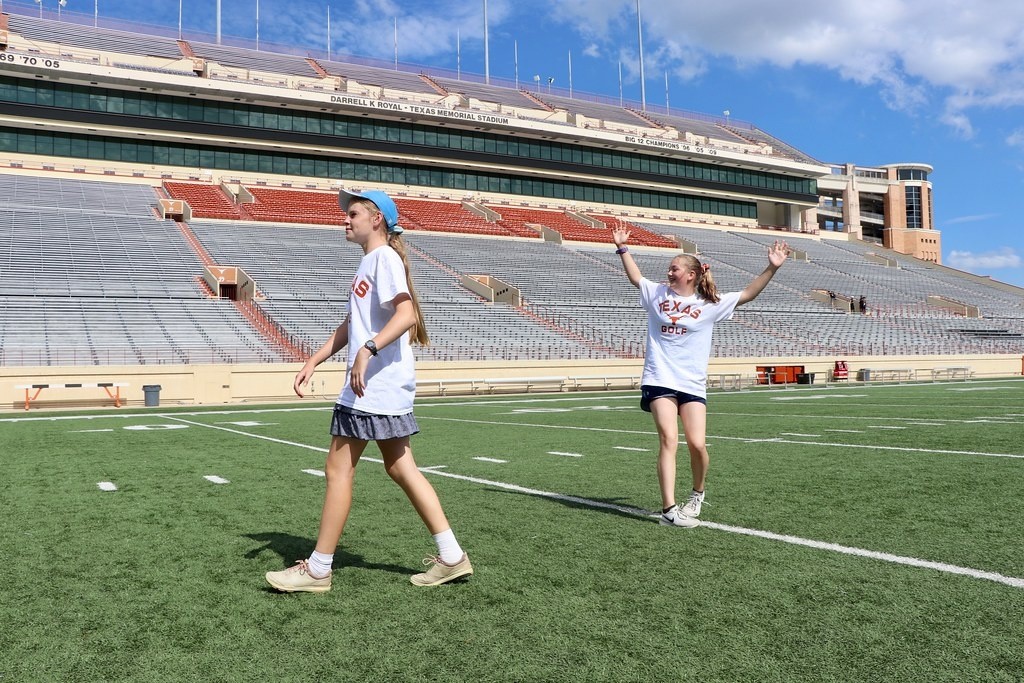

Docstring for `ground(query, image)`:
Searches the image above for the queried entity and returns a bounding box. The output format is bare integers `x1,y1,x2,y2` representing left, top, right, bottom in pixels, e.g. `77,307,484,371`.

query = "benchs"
0,173,1024,409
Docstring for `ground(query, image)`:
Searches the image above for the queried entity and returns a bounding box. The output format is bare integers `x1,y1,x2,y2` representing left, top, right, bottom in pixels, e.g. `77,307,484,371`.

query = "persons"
611,219,790,528
828,291,836,298
850,297,855,312
265,189,473,592
860,295,866,312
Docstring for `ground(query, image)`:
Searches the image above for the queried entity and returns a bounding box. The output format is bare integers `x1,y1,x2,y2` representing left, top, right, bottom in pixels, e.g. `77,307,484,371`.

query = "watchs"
364,340,379,357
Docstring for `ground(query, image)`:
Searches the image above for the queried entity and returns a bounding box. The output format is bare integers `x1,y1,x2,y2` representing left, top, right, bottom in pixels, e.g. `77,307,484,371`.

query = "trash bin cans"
796,372,815,384
756,365,805,384
142,384,162,406
861,368,870,381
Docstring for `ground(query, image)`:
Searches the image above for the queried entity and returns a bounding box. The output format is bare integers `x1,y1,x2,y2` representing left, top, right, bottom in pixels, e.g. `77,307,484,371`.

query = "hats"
338,188,398,235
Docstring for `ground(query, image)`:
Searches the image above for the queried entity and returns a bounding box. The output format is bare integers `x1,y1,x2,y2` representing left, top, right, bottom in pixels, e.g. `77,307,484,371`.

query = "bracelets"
616,247,628,254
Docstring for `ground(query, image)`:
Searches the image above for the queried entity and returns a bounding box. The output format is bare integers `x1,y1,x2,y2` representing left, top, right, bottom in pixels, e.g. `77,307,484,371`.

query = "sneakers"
265,559,332,593
681,488,705,518
409,550,474,587
659,502,700,529
